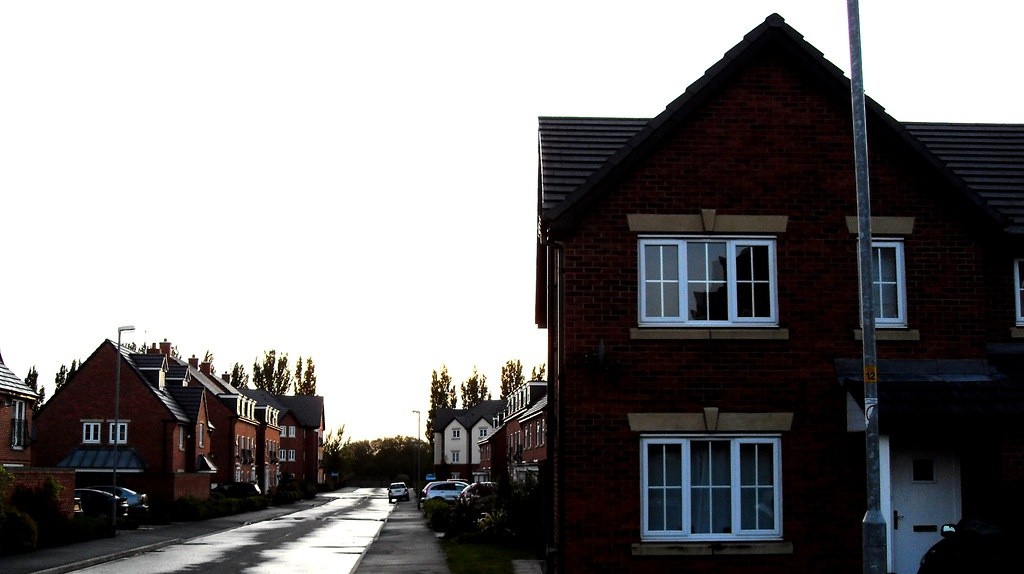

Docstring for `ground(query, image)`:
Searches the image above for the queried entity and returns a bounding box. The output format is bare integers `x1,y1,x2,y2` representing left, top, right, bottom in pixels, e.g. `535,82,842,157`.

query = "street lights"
108,325,138,537
413,410,422,506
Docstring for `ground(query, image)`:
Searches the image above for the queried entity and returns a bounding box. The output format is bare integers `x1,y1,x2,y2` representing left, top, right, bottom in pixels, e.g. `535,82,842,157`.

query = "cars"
421,479,471,506
76,487,149,528
73,488,129,528
212,481,262,498
456,481,498,503
387,480,410,503
74,497,85,521
